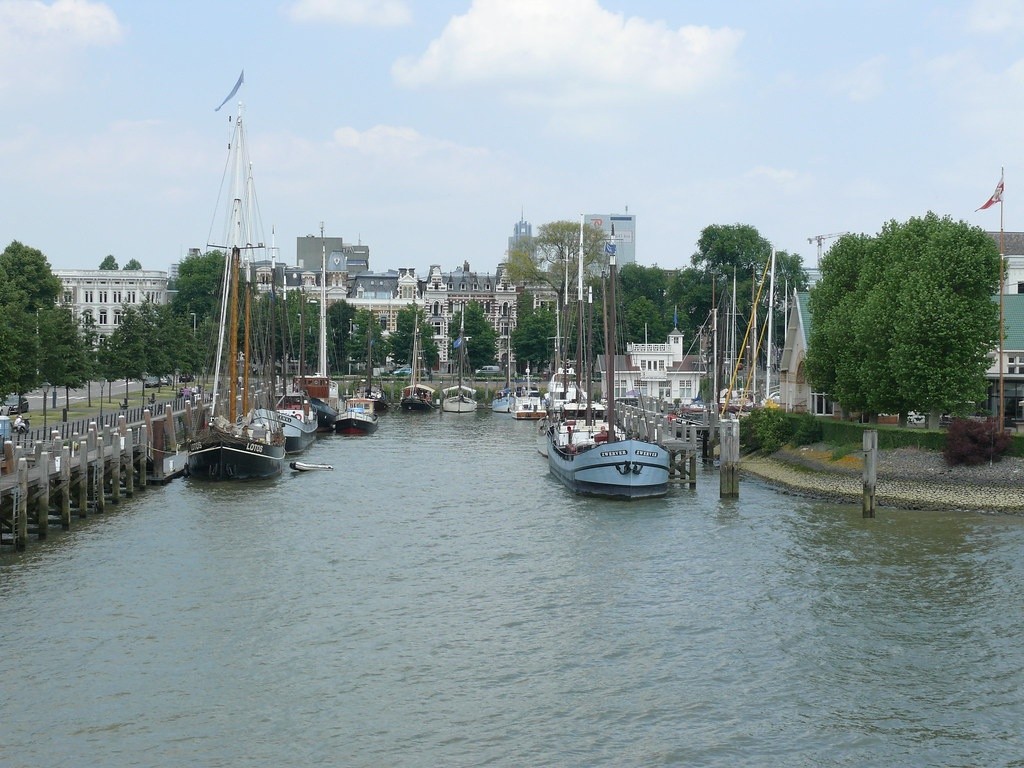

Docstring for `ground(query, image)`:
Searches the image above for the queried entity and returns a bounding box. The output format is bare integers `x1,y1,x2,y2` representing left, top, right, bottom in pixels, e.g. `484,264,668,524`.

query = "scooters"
17,414,32,435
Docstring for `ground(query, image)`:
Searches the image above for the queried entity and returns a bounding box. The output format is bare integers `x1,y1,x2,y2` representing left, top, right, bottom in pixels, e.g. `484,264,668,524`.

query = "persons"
13,415,23,430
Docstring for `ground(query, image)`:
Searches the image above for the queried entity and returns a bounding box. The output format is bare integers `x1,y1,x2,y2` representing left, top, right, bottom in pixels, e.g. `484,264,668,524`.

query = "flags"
974,175,1004,213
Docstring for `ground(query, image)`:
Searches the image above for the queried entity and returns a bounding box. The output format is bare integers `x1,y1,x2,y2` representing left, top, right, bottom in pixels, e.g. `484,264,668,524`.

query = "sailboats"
246,219,391,459
440,301,478,413
490,302,516,413
399,313,438,411
536,212,794,464
186,99,287,486
544,222,675,504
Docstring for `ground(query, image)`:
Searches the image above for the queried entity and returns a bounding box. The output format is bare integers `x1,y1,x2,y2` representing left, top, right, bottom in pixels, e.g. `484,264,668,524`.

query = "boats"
508,358,546,420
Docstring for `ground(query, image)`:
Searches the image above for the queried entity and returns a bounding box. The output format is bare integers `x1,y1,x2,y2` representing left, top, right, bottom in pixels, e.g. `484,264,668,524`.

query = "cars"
408,368,431,377
0,394,30,416
145,374,169,389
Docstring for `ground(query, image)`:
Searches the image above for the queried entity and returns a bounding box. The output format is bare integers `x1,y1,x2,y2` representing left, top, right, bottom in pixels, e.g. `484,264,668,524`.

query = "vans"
475,365,501,376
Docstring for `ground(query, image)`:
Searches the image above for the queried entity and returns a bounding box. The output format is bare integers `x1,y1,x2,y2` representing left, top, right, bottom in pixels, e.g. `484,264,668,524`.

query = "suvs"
392,366,412,377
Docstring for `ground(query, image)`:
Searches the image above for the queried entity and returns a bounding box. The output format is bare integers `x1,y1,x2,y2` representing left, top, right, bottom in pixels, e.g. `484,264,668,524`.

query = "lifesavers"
295,414,302,421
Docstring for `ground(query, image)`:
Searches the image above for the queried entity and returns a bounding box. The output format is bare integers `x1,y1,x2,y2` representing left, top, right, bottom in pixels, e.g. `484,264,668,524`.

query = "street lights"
41,377,50,443
174,366,180,413
97,372,106,433
142,369,148,421
